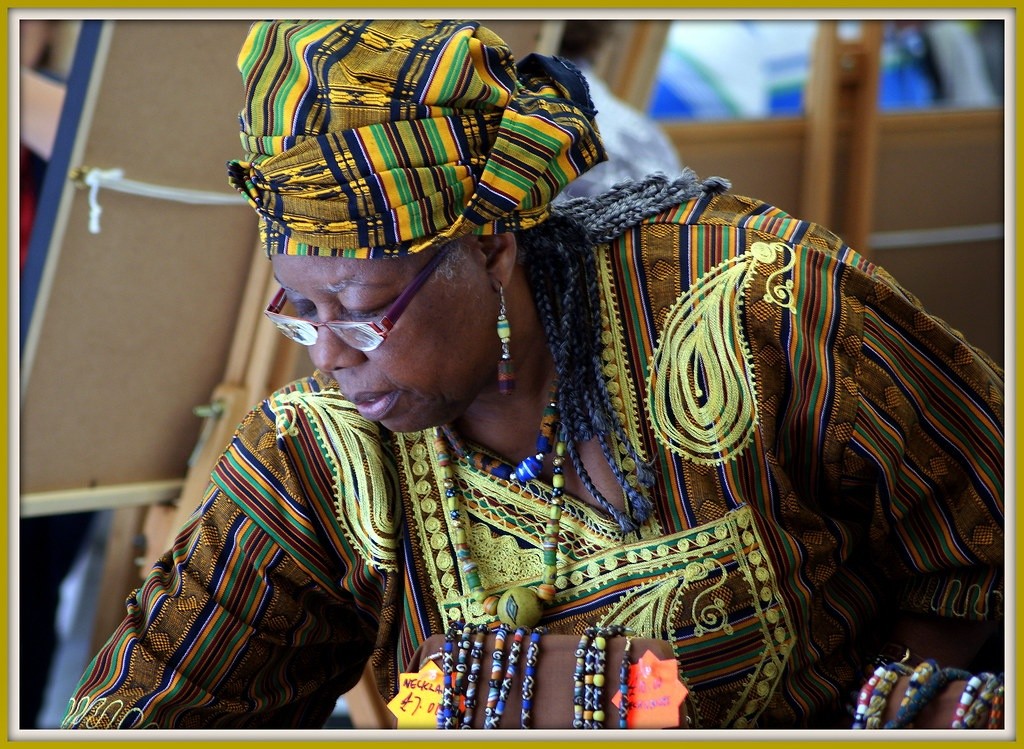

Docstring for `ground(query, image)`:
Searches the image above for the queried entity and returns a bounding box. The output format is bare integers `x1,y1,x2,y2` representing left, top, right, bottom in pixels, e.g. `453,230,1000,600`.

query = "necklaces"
430,374,570,629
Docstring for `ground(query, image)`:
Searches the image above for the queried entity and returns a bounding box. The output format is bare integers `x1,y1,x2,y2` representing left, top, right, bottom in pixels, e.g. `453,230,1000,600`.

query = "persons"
55,19,1004,730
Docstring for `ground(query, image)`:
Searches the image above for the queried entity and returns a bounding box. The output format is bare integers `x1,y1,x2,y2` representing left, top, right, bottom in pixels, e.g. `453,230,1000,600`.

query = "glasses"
263,240,459,352
